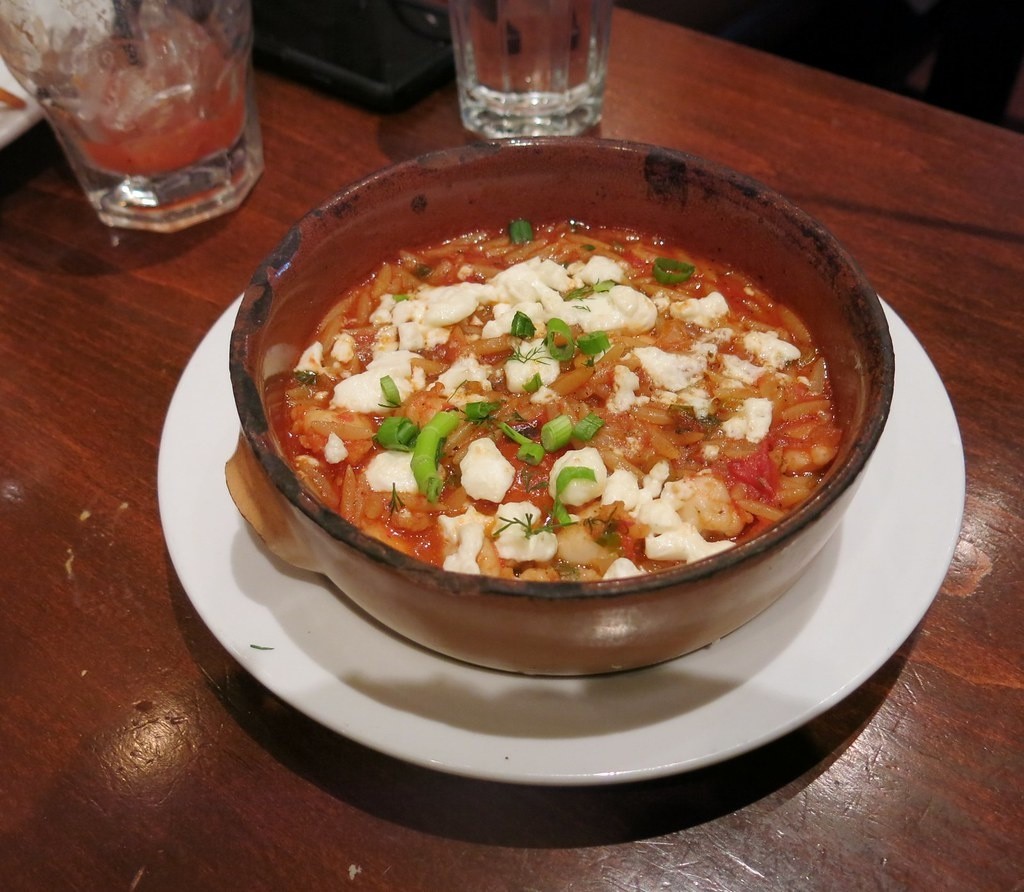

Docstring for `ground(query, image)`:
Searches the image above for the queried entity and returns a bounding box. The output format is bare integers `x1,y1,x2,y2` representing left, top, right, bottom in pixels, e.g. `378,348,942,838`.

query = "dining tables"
0,1,1024,892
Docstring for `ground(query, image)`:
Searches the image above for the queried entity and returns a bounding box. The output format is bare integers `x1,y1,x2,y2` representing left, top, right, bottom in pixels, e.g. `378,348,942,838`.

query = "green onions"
378,219,696,527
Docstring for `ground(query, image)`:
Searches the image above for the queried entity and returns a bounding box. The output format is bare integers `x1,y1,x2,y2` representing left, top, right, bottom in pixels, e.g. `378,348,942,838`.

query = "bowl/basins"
224,136,899,679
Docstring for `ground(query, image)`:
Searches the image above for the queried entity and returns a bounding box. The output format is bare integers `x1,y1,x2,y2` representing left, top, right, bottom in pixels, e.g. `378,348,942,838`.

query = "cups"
448,0,613,139
0,0,264,232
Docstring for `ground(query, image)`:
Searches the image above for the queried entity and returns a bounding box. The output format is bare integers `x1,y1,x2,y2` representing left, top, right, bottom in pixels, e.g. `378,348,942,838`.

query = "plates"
157,293,966,786
